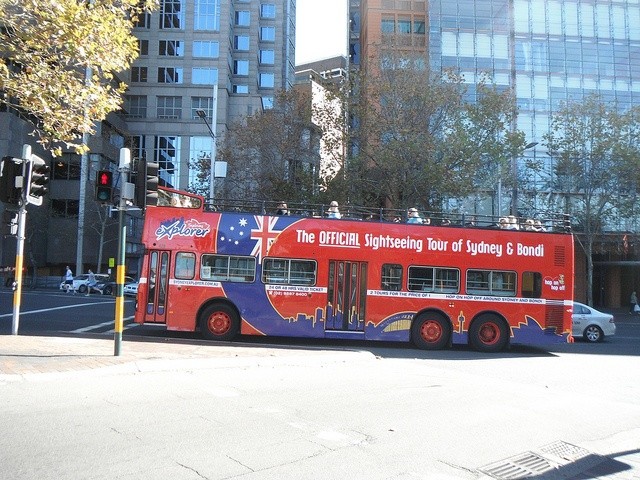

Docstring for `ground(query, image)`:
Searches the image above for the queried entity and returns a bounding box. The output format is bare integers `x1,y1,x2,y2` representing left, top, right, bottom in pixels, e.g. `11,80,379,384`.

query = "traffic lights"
134,151,160,208
37,163,50,195
95,169,113,203
27,155,45,206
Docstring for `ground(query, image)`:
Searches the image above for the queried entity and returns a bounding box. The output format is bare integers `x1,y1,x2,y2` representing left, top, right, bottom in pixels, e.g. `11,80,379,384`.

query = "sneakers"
101,290,103,294
85,294,89,297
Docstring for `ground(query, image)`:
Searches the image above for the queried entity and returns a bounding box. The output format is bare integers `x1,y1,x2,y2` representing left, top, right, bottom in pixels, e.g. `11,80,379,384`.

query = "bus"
132,186,575,351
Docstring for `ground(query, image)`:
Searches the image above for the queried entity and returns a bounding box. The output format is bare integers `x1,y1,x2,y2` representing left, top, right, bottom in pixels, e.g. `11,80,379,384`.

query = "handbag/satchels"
634,304,640,312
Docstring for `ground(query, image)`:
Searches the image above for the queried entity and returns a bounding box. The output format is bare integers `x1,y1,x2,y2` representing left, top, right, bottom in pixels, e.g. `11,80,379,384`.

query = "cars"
91,274,137,295
572,301,616,342
123,282,139,297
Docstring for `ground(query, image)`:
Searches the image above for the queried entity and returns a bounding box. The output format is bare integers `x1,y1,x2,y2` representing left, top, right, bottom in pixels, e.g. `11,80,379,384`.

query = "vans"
60,272,109,293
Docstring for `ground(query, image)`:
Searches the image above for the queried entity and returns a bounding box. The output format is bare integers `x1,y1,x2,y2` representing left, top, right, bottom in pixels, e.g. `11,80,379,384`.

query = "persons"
327,200,341,219
534,219,544,231
524,219,536,231
628,289,638,314
83,267,104,297
506,214,518,230
405,207,423,225
271,200,289,214
63,265,78,295
498,218,507,229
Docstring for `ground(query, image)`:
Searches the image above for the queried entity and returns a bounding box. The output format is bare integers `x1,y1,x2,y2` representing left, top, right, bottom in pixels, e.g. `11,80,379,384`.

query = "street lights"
195,108,223,209
496,142,539,215
